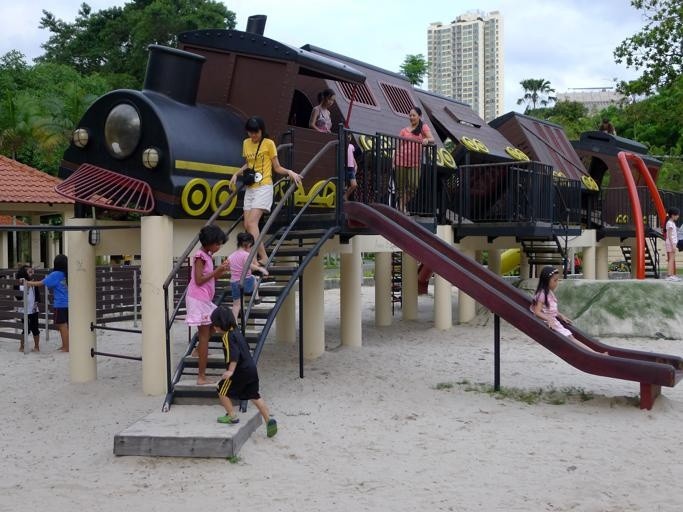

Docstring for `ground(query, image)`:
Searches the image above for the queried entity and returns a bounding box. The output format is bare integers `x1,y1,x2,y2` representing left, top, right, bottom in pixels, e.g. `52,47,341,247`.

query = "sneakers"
665,275,681,281
218,413,240,423
266,417,277,437
255,296,261,304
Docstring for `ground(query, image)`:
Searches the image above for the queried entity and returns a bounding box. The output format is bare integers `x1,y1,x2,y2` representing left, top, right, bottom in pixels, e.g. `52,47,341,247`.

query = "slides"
344,201,683,411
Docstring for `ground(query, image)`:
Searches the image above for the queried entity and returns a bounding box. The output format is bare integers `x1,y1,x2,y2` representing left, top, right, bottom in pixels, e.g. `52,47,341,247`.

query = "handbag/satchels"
242,167,255,187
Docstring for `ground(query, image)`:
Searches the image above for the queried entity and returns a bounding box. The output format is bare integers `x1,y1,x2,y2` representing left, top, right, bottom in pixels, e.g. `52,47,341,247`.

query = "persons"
665,207,682,277
529,266,610,357
224,231,270,328
185,224,232,386
14,265,42,354
391,106,435,216
228,115,305,269
309,90,337,135
211,306,279,439
599,118,617,138
28,254,70,354
344,132,359,203
